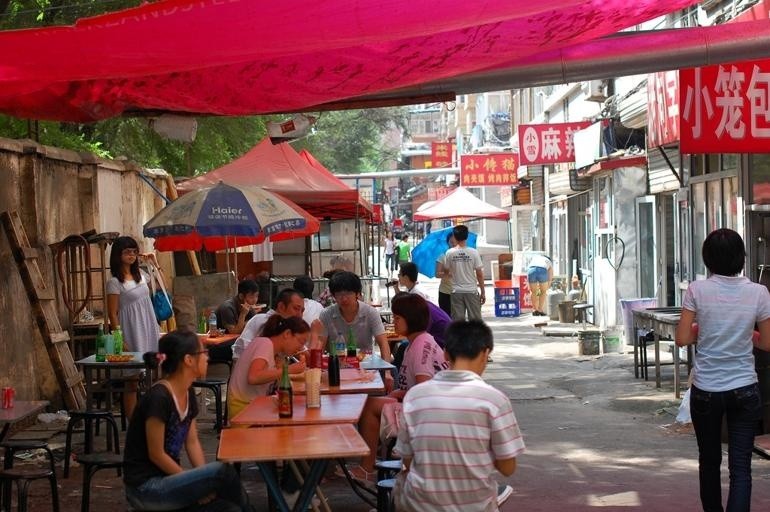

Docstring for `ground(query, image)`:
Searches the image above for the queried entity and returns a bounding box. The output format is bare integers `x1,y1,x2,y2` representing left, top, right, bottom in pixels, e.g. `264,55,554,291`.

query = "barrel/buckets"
493,279,513,295
618,298,659,345
577,330,600,355
558,300,575,323
601,330,623,353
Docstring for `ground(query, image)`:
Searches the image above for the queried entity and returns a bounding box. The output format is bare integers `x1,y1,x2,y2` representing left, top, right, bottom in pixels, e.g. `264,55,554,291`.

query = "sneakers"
532,310,546,316
280,489,328,511
497,485,514,506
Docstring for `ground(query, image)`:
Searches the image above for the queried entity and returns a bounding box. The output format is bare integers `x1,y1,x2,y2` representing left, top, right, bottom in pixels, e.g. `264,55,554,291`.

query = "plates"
251,303,267,308
106,354,135,362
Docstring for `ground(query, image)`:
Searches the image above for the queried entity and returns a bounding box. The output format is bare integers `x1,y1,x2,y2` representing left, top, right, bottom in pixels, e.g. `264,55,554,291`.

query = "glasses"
189,349,209,355
334,293,353,300
122,250,138,255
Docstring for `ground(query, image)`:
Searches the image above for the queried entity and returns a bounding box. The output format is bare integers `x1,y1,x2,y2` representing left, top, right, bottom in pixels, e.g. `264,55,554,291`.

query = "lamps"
267,110,320,145
149,113,198,142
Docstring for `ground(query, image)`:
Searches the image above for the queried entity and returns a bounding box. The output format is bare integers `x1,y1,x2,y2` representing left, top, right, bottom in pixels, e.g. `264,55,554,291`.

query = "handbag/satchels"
151,289,173,321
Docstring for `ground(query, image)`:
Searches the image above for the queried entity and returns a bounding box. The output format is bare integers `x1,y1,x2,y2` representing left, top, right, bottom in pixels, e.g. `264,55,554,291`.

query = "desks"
632,307,697,399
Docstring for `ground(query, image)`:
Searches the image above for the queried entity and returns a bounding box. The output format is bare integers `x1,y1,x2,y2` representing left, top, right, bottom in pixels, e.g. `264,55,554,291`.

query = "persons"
677,228,770,512
443,223,495,363
389,318,525,512
391,290,453,347
378,227,412,281
105,234,160,423
225,315,322,431
434,232,456,317
121,328,251,512
391,262,438,306
328,293,450,489
214,267,393,372
527,254,554,316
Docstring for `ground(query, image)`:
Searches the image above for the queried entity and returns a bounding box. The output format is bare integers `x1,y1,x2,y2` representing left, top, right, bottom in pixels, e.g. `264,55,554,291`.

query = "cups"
105,335,114,353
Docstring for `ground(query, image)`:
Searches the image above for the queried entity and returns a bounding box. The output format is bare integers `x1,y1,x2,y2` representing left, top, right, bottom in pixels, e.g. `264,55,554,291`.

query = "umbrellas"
408,225,478,280
152,190,321,293
142,178,305,298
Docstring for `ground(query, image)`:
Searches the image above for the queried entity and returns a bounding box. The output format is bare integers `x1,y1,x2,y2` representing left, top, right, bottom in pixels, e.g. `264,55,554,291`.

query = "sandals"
334,464,377,484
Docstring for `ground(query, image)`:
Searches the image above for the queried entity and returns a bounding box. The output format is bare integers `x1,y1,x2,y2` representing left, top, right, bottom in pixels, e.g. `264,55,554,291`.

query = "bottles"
328,340,340,386
209,310,217,337
347,326,356,356
198,313,206,333
95,323,105,361
310,349,323,369
114,325,123,354
336,332,345,356
278,364,292,418
385,280,399,287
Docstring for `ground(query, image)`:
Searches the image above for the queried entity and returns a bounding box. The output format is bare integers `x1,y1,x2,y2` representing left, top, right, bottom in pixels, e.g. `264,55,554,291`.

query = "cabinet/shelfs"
63,238,111,360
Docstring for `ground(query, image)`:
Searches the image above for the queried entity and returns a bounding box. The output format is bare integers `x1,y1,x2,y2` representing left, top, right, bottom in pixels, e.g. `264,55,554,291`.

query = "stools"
64,411,122,478
573,304,594,330
375,461,401,481
193,379,226,428
376,479,395,510
4,442,54,473
77,454,124,512
1,469,57,511
